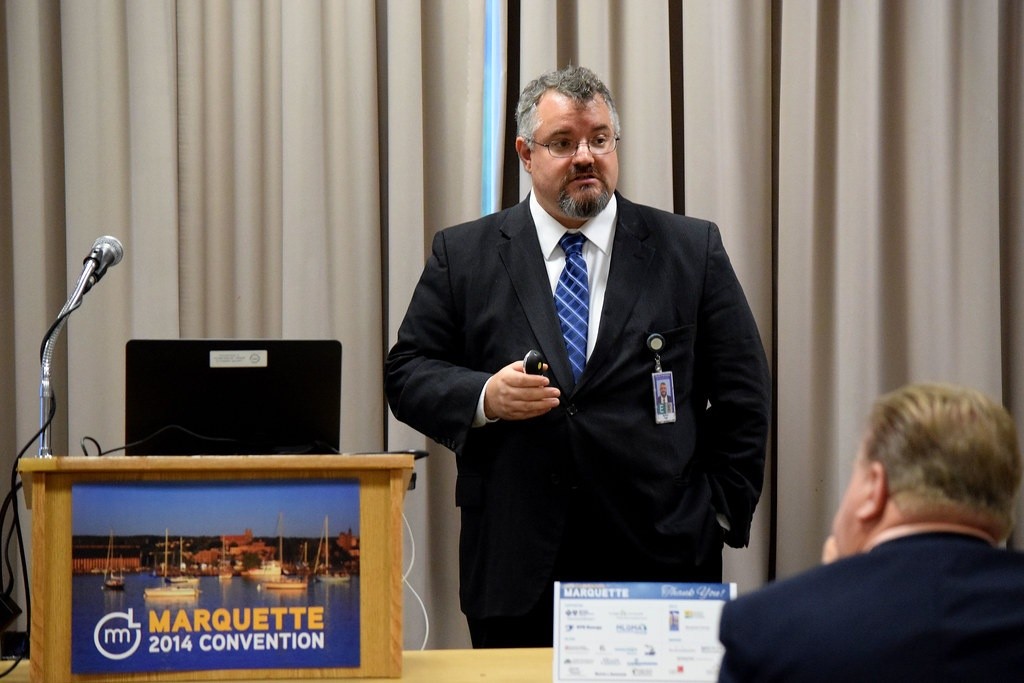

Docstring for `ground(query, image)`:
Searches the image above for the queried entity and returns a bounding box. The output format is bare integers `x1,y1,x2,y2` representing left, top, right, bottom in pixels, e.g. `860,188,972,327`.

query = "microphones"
78,235,123,294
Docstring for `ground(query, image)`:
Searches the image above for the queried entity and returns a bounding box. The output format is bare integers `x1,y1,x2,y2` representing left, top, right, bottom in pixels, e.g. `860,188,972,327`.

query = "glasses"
523,133,621,158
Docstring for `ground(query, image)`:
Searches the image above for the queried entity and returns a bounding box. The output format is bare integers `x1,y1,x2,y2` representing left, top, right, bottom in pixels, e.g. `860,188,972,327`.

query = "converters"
0,592,22,633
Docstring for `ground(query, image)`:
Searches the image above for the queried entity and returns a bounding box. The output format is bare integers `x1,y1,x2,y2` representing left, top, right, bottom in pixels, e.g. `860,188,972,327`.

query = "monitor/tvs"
125,339,342,454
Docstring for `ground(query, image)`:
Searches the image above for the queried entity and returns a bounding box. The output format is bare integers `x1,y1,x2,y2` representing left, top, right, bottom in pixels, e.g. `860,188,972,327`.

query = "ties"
553,231,590,385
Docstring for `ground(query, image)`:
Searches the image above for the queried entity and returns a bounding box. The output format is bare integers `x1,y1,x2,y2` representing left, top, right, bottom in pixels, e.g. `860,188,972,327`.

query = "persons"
656,381,673,404
385,64,777,654
723,380,1024,683
